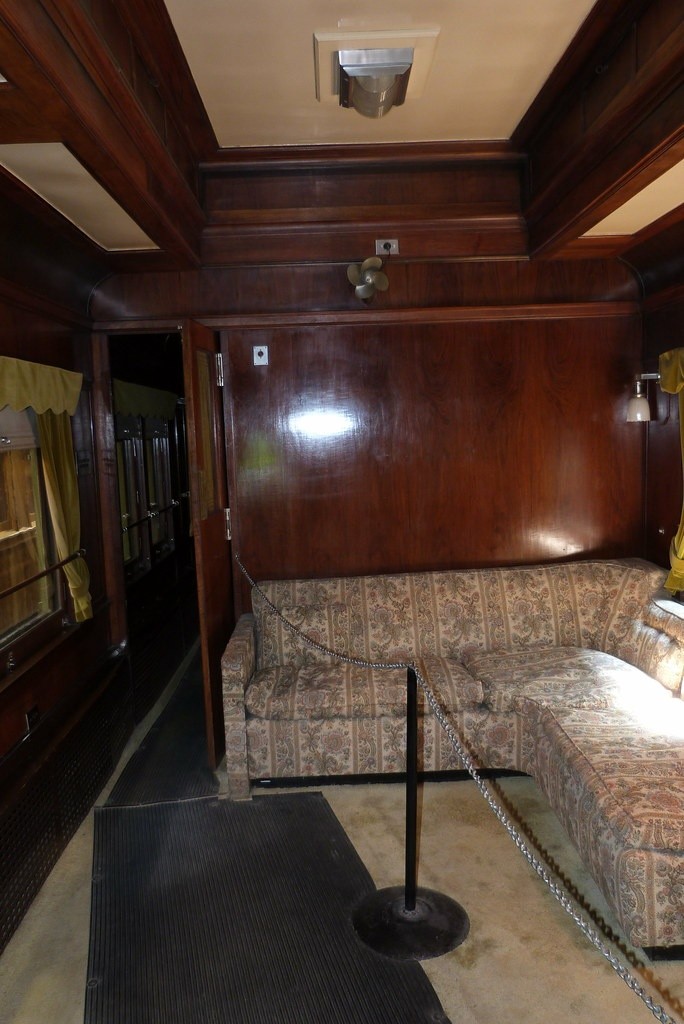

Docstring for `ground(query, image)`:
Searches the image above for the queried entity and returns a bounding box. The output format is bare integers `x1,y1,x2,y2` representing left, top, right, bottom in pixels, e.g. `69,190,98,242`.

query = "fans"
346,257,389,299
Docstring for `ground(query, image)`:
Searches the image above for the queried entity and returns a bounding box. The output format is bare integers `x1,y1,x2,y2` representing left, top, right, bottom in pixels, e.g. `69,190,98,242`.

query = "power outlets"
253,346,268,366
375,240,399,255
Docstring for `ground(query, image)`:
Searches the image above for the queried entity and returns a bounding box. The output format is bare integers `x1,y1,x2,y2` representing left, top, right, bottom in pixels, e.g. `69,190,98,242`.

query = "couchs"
218,557,684,962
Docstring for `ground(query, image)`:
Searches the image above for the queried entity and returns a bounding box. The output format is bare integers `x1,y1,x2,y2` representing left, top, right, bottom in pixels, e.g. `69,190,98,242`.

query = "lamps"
313,25,438,118
624,373,670,425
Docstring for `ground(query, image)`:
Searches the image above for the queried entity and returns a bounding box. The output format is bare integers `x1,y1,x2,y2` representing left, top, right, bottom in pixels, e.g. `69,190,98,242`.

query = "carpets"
0,598,452,1024
